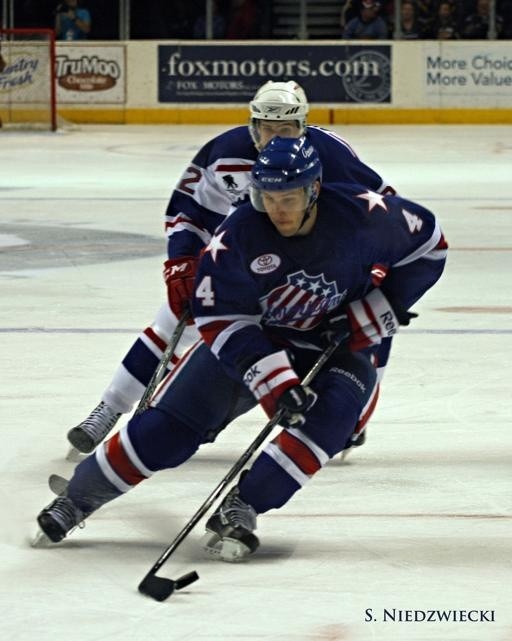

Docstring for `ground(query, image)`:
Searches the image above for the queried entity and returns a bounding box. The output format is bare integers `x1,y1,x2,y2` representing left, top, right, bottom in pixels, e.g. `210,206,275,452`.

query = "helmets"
249,135,322,212
248,80,309,153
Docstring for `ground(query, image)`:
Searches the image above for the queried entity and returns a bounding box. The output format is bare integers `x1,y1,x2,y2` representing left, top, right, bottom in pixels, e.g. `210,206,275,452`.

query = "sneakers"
206,485,259,553
68,400,123,453
37,490,95,542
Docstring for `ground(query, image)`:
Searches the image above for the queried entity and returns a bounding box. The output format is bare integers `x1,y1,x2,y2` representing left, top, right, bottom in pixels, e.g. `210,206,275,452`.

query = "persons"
31,134,448,562
336,0,511,42
52,1,92,41
67,78,402,461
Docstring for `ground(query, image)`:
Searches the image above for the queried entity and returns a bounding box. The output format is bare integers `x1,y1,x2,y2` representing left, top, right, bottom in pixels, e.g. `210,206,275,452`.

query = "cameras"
52,4,69,15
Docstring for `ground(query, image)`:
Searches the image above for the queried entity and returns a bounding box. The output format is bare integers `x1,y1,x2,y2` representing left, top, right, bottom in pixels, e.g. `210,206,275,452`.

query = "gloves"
243,350,319,428
319,285,410,356
163,256,199,325
